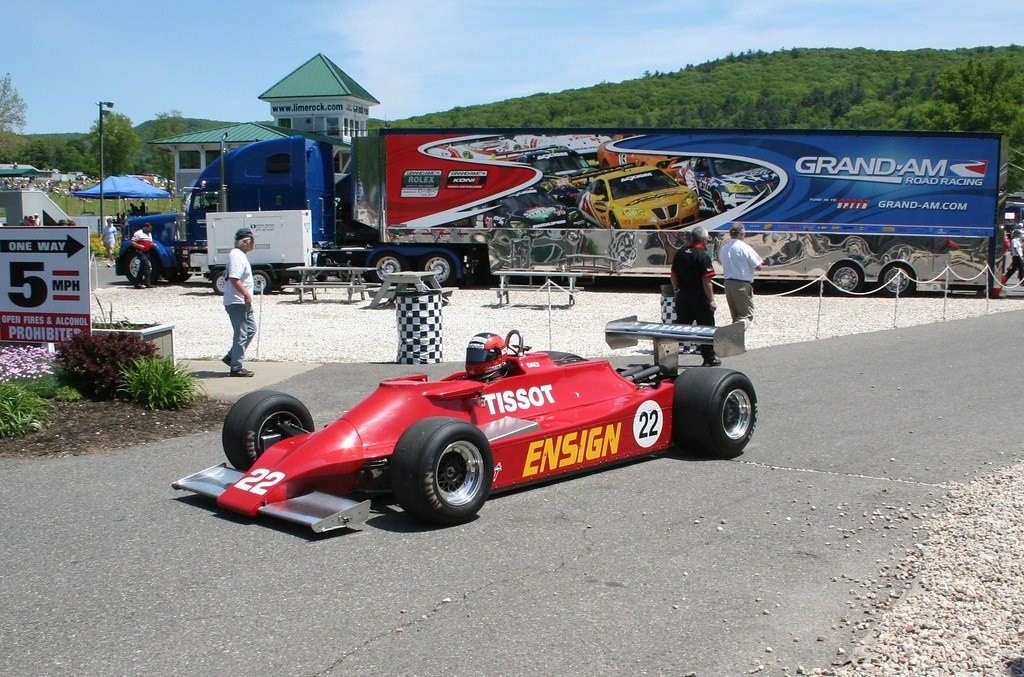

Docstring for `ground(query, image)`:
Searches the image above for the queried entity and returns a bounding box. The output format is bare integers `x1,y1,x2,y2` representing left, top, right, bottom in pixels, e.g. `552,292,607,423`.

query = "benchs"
492,283,589,293
368,285,453,298
281,277,366,303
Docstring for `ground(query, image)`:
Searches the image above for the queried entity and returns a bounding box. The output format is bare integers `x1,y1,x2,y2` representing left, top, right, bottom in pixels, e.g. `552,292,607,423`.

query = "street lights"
97,101,116,233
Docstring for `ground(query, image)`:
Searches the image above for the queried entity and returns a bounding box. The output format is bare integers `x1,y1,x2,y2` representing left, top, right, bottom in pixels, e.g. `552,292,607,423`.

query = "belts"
726,278,750,283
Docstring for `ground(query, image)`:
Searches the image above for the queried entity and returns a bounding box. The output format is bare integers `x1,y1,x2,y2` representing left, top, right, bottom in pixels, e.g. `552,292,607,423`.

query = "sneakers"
702,358,721,366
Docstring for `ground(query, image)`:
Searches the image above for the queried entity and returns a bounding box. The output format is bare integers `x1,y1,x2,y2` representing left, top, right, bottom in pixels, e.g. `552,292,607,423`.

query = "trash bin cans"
395,288,442,363
661,285,698,353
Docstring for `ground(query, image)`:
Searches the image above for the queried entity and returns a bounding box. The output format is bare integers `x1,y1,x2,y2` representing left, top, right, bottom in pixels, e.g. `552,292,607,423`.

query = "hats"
234,228,259,240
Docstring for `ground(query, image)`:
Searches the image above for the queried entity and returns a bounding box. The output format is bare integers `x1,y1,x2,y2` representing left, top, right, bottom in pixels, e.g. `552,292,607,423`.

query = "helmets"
465,332,507,375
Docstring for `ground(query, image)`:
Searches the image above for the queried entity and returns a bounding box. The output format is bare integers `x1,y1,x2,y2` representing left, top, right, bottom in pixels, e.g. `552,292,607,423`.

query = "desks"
493,271,584,305
370,271,450,310
285,265,368,302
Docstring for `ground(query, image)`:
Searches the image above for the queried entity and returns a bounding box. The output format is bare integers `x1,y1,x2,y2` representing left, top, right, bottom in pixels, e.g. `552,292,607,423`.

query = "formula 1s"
169,314,757,540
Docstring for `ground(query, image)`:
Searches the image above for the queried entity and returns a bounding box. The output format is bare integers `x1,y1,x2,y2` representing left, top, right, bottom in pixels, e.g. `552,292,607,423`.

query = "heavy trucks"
179,127,1009,297
115,174,358,286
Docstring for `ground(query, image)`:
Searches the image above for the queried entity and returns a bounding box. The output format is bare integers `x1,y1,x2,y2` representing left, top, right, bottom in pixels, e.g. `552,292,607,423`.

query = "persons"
102,218,118,267
670,227,722,367
0,175,175,202
722,223,764,331
465,333,509,384
24,213,76,226
222,228,258,377
1002,223,1024,286
131,223,158,289
109,202,148,223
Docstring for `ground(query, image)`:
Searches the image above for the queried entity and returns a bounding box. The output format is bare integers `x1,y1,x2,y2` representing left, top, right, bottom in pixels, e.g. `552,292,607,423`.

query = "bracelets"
673,288,681,293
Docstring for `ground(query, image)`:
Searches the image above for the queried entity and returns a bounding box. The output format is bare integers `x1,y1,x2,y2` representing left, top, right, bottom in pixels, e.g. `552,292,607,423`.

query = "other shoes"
134,285,145,289
145,284,157,288
222,355,231,367
230,368,254,376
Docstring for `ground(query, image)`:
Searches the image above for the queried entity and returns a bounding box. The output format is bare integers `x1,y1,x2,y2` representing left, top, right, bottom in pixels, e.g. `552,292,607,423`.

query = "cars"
490,144,780,232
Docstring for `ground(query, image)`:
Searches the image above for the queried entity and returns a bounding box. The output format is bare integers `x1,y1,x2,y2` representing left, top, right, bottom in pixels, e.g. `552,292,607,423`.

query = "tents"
0,168,52,189
72,176,172,218
